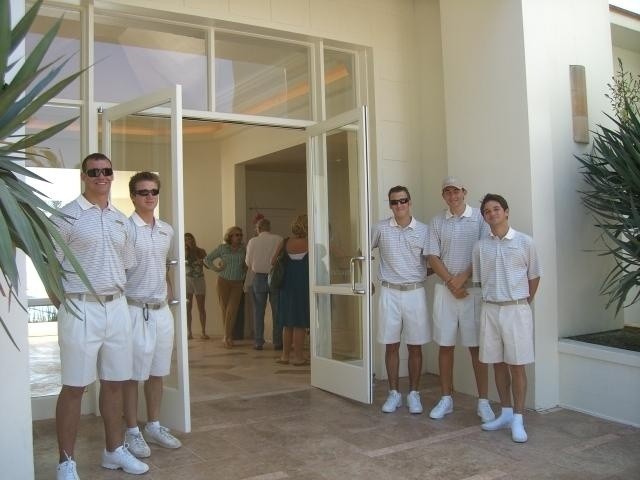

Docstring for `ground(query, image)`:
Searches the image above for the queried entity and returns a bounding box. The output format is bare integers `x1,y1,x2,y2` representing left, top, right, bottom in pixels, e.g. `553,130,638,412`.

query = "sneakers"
57,460,80,480
101,445,149,475
511,413,529,443
382,389,404,412
141,423,182,449
480,406,514,431
428,394,454,418
405,390,423,414
477,397,496,422
124,431,152,458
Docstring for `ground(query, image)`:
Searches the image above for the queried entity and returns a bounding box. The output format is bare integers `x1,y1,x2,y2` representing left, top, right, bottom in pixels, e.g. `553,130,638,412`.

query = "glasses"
133,189,160,196
84,168,112,177
390,197,408,206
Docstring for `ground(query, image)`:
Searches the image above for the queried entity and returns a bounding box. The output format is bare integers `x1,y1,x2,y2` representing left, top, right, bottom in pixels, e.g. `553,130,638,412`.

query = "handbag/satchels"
267,237,290,295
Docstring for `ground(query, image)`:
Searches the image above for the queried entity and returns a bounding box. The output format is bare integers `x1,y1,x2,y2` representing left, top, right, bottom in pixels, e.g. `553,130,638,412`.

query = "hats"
440,174,469,195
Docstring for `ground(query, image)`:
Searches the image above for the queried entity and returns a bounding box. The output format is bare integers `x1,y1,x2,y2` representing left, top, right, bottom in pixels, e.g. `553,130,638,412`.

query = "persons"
471,193,543,443
41,152,153,480
182,209,309,367
367,185,437,415
111,171,182,459
418,174,498,427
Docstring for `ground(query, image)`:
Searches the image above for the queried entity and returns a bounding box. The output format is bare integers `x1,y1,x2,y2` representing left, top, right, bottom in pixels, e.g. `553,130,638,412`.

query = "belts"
434,279,482,289
64,290,123,302
483,297,527,306
381,279,425,292
125,296,168,310
